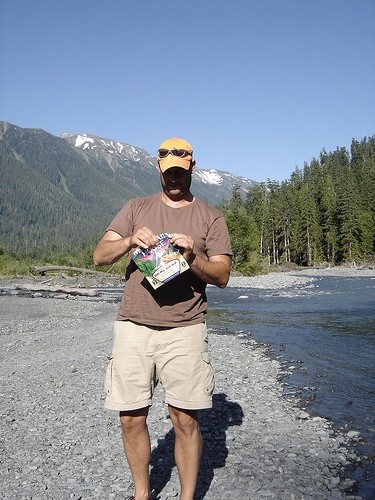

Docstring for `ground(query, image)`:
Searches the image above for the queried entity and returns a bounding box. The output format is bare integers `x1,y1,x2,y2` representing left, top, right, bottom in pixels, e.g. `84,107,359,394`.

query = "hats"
158,137,193,173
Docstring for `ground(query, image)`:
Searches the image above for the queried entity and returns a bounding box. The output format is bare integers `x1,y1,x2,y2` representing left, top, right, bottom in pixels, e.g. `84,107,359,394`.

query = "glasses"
156,148,191,158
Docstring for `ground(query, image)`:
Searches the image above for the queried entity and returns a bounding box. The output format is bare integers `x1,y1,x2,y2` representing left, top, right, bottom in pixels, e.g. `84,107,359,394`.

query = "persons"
93,137,235,500
